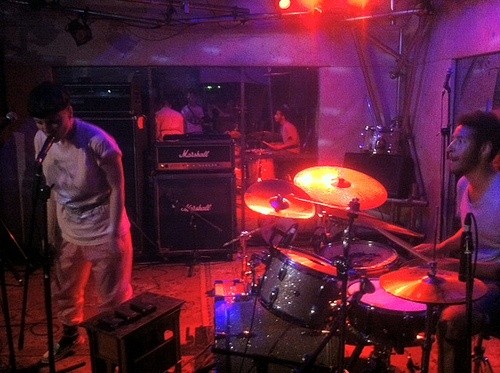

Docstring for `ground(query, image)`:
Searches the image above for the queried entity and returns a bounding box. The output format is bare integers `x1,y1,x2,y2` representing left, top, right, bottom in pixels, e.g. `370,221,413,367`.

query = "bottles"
358,118,401,154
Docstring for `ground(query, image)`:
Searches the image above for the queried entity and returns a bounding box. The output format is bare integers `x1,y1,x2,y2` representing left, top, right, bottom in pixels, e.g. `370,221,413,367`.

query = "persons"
156,89,205,137
411,110,500,373
269,108,303,153
29,81,133,365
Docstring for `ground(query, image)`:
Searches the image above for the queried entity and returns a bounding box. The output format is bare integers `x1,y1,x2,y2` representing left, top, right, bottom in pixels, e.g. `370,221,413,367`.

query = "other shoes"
40,335,83,364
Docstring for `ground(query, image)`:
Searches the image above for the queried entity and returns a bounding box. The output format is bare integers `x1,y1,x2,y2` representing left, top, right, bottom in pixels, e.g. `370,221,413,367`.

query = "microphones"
458,214,474,281
32,134,56,171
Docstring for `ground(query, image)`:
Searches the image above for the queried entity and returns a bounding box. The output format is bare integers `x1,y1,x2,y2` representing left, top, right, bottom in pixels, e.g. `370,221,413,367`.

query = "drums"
342,277,437,346
244,155,277,185
257,243,339,331
318,239,402,276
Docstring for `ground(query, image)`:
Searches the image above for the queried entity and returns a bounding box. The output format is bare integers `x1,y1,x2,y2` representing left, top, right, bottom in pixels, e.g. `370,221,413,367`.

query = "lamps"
273,0,291,11
66,20,92,46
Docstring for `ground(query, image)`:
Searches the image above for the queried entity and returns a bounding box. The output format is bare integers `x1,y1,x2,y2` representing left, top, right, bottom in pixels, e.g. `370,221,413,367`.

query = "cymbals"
242,178,316,220
250,130,277,141
315,203,426,240
243,148,274,154
293,165,388,211
379,265,493,306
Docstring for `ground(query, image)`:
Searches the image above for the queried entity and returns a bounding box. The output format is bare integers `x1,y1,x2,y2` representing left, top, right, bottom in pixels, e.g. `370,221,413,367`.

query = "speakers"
69,113,148,260
152,172,238,259
81,291,187,373
342,152,414,198
